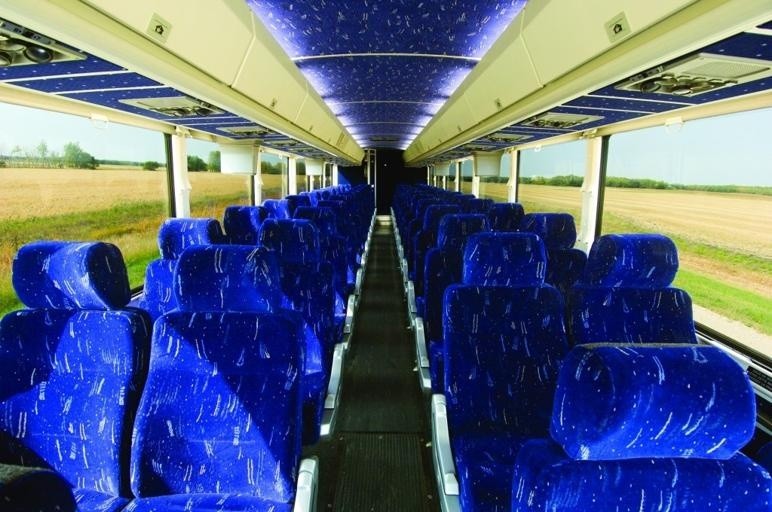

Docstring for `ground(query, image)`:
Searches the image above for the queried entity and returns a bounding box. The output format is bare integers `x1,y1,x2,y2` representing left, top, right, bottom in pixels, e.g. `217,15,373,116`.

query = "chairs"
0,176,379,512
385,172,772,512
